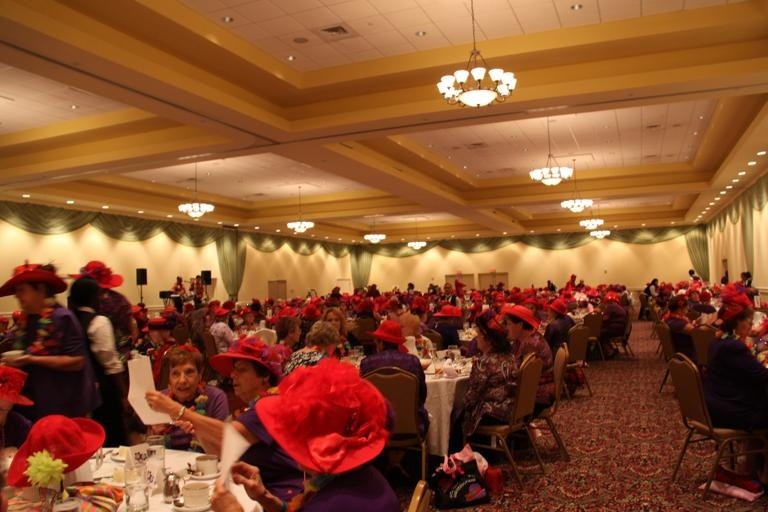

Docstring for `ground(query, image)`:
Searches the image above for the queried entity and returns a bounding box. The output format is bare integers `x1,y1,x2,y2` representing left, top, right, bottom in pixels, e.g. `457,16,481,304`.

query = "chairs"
157,293,768,512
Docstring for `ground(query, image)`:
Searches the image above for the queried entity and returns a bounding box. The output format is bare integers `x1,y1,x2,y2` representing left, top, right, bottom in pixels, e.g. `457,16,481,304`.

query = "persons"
0,262,103,421
0,269,628,512
642,268,768,491
69,262,148,445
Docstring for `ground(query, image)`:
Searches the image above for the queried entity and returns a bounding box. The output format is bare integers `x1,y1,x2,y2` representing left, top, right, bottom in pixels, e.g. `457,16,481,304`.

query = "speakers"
201,270,211,284
136,268,147,285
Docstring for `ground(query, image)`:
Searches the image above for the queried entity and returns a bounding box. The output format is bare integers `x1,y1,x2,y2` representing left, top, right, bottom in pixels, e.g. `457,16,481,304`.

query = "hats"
254,355,388,476
69,260,124,289
0,264,68,298
0,364,36,407
133,284,626,330
648,279,760,335
208,333,289,378
5,414,106,488
196,274,202,280
366,319,406,345
473,315,510,347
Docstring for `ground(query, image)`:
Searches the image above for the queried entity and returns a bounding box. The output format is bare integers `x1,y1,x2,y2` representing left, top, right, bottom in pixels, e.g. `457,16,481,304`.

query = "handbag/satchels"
438,444,489,478
428,458,492,509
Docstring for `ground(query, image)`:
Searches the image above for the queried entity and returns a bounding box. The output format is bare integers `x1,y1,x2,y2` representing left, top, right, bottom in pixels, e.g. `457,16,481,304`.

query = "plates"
109,449,126,462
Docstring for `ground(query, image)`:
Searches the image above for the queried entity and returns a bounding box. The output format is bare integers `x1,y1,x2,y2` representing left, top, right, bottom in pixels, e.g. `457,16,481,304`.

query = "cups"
119,436,231,512
426,339,473,379
350,344,365,367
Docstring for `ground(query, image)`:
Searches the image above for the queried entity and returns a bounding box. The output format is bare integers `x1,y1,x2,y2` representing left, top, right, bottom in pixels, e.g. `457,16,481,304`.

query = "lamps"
286,187,315,232
178,163,215,218
363,217,386,244
407,219,426,250
435,0,518,108
529,117,611,240
